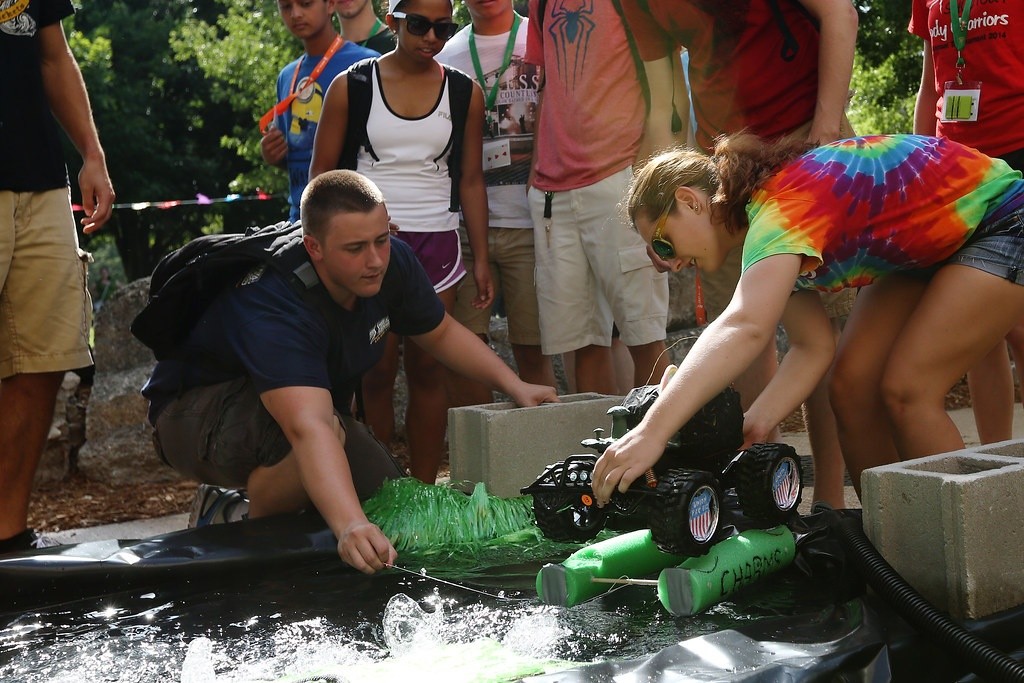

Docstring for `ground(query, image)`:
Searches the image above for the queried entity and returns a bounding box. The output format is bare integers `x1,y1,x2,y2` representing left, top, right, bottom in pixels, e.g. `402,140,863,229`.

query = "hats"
389,0,454,14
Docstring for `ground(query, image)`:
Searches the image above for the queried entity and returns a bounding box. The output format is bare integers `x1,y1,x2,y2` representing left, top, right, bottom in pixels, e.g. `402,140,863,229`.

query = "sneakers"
188,483,245,529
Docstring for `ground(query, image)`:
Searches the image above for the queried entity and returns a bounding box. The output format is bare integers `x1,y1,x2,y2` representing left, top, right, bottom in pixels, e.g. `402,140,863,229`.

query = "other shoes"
811,501,833,516
30,536,60,548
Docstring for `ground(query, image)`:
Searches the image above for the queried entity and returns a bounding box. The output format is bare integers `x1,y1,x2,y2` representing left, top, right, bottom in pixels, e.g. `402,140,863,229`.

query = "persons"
307,0,495,484
259,0,397,227
131,168,562,577
0,0,116,556
908,1,1023,446
527,0,671,394
617,0,856,509
434,0,558,407
590,128,1024,509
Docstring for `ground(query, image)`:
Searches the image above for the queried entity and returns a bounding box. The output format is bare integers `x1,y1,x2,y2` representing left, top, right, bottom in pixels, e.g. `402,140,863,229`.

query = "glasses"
392,12,458,40
652,194,676,262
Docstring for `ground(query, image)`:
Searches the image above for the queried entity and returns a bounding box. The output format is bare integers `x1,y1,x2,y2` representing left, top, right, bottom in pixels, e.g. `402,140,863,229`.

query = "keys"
545,224,551,248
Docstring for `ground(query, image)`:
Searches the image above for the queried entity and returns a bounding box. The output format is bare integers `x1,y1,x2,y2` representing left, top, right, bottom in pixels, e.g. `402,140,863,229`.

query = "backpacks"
130,218,411,376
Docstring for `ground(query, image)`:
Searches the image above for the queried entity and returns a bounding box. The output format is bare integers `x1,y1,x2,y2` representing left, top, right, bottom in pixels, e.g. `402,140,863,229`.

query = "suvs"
521,376,805,559
